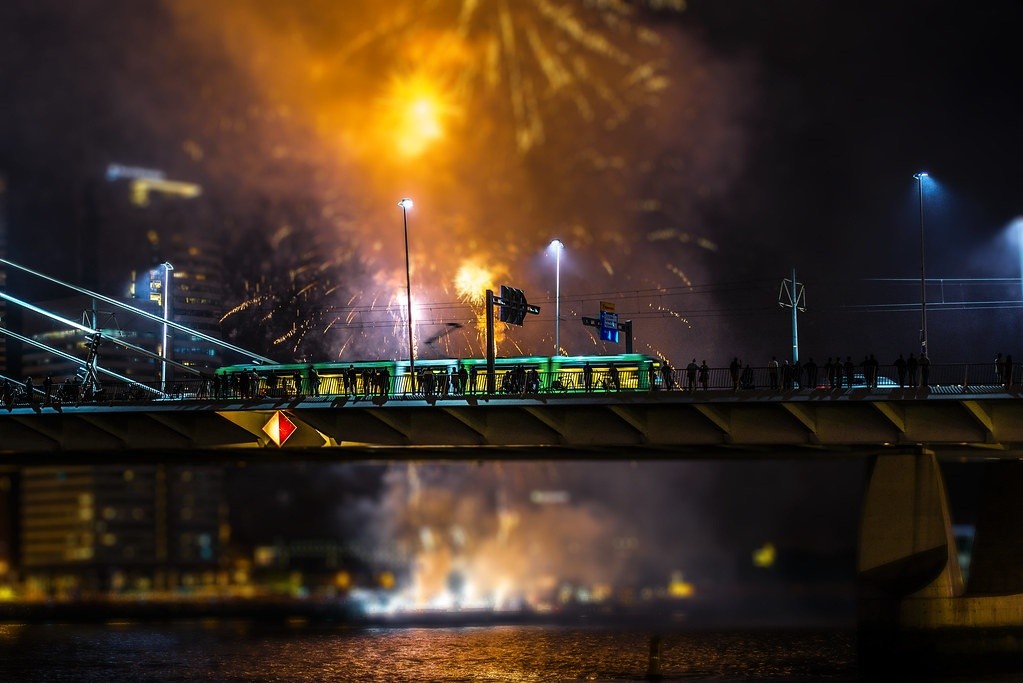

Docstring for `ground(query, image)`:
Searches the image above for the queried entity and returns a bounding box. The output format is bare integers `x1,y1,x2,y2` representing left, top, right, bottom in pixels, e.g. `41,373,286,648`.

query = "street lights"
398,197,419,399
549,239,562,356
914,170,929,388
161,262,173,392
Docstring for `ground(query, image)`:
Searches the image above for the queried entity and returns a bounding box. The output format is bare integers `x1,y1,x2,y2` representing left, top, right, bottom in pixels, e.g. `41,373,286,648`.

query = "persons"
3,379,11,394
63,376,81,396
266,369,278,388
730,357,753,388
892,351,931,387
608,363,620,392
214,368,259,400
648,363,656,386
994,352,1012,386
503,364,543,393
662,361,673,391
343,364,390,396
767,353,878,390
687,358,710,390
293,369,302,394
43,376,53,394
308,365,318,394
26,377,33,395
417,364,477,395
584,361,593,393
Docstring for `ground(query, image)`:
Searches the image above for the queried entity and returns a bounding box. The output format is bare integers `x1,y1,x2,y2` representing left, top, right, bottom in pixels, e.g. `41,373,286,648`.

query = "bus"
211,353,662,395
